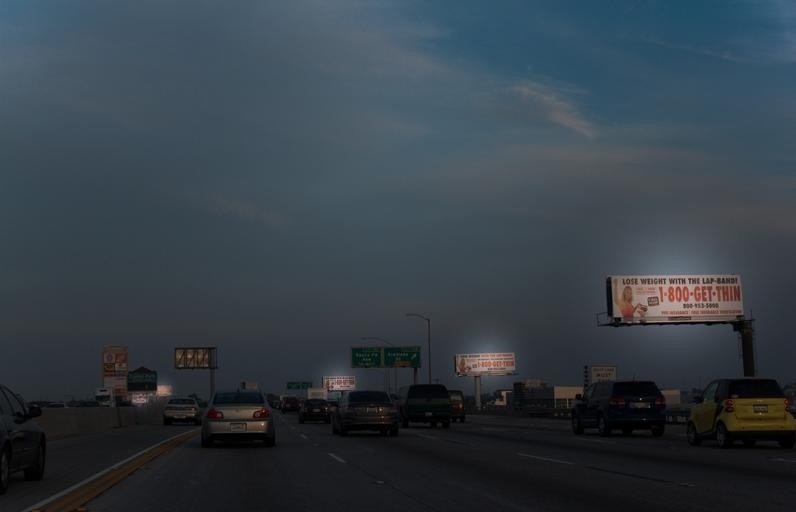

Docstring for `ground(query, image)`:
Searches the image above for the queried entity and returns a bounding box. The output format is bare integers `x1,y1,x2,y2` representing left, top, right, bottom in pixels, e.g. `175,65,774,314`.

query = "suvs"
447,389,466,423
162,398,202,427
569,380,667,436
398,383,450,429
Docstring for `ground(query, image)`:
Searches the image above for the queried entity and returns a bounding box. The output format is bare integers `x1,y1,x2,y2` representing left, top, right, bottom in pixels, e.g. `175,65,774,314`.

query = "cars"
267,390,404,437
199,387,276,449
0,384,47,496
686,375,796,450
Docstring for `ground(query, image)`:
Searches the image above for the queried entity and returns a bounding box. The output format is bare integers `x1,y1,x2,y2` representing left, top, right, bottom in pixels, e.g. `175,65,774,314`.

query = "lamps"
736,315,745,321
614,317,621,322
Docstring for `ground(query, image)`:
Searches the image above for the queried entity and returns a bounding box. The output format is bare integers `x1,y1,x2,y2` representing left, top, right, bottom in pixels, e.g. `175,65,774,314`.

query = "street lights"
403,312,431,384
360,336,397,392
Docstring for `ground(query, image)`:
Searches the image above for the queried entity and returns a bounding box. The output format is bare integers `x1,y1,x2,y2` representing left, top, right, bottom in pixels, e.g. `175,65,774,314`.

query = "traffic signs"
383,345,421,369
351,347,382,369
